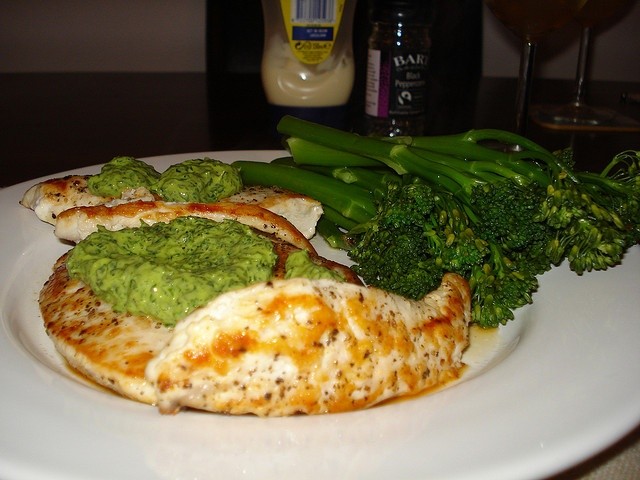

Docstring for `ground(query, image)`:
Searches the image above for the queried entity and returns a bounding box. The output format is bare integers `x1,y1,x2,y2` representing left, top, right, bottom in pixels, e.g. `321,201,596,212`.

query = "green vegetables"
231,113,640,329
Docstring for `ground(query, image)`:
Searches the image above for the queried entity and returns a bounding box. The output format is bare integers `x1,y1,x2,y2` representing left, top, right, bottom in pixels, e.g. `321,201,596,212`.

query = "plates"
0,150,639,479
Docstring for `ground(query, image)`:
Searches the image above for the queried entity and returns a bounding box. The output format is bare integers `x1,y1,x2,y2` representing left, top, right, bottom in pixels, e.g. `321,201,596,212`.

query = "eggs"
17,157,324,244
37,224,471,416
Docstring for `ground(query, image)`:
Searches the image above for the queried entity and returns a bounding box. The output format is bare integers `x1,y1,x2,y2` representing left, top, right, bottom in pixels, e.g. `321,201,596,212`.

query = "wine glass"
480,0,588,155
543,0,637,126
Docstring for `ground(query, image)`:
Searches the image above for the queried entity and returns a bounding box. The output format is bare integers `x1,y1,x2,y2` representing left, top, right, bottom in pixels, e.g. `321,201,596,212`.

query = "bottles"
353,6,435,136
